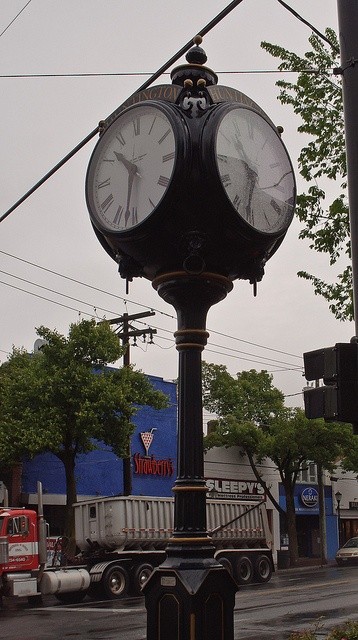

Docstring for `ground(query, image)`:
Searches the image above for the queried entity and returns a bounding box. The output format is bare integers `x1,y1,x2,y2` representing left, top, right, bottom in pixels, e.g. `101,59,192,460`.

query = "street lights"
334,491,342,548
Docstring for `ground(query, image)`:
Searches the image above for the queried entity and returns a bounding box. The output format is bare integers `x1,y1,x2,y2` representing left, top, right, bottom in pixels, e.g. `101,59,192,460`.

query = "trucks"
0,478,275,602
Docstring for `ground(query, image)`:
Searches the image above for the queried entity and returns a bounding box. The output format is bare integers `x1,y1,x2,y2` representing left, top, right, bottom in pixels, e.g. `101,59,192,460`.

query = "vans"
334,536,358,567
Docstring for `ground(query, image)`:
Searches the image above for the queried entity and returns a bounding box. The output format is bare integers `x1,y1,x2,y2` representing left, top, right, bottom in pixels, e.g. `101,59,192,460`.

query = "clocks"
214,108,295,233
86,106,172,228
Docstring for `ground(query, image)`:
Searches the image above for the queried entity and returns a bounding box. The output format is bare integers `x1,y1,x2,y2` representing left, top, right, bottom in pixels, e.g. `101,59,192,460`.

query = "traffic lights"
302,343,357,423
